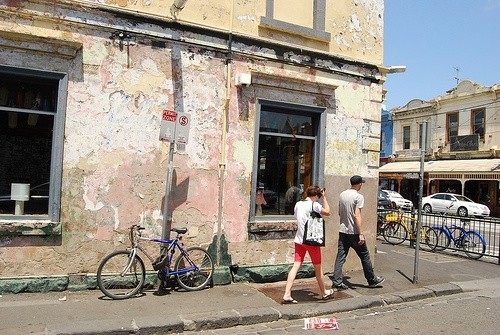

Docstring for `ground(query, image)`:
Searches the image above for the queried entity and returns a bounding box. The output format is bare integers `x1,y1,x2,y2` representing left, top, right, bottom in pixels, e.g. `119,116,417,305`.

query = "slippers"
322,288,337,299
281,297,298,303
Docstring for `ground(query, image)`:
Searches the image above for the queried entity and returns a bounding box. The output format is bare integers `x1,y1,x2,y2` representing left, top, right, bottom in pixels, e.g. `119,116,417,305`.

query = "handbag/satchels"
302,201,325,247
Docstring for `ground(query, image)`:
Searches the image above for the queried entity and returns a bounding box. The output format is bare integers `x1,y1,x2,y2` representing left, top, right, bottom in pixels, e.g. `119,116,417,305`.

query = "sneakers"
332,282,350,290
369,276,385,287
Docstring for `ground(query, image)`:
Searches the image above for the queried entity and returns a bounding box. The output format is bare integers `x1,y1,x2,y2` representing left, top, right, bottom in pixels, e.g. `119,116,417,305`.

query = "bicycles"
424,204,488,260
96,224,215,300
377,207,439,252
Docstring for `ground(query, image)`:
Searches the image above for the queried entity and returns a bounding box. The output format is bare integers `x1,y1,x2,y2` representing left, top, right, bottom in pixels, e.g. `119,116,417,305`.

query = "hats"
349,174,366,184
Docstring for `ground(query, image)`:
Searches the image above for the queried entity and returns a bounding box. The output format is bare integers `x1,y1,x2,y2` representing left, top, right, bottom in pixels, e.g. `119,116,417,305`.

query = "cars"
0,182,50,214
381,189,413,211
256,182,278,205
377,189,393,211
422,192,491,219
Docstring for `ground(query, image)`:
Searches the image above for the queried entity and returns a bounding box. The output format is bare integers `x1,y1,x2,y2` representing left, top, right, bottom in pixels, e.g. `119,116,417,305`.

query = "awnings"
379,159,500,172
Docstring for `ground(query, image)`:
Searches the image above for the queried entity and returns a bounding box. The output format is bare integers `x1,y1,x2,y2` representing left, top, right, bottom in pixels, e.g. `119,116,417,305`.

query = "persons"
284,181,304,213
333,175,385,290
282,185,335,304
